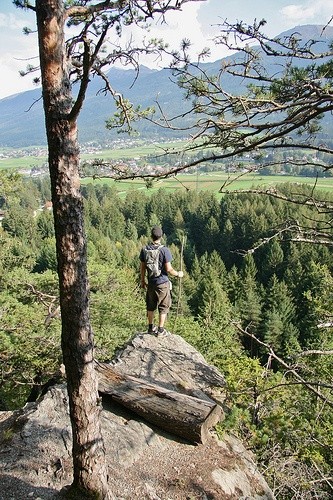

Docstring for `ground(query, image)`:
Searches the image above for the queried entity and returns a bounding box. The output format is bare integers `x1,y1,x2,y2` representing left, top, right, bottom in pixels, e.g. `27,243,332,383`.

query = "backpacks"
142,245,165,279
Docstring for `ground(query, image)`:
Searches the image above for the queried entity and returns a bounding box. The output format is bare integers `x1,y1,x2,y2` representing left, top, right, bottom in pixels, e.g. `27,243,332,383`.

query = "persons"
138,227,184,338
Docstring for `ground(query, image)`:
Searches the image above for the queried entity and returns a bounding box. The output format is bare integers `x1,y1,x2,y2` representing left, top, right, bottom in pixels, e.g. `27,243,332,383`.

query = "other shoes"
148,326,157,334
156,330,171,337
